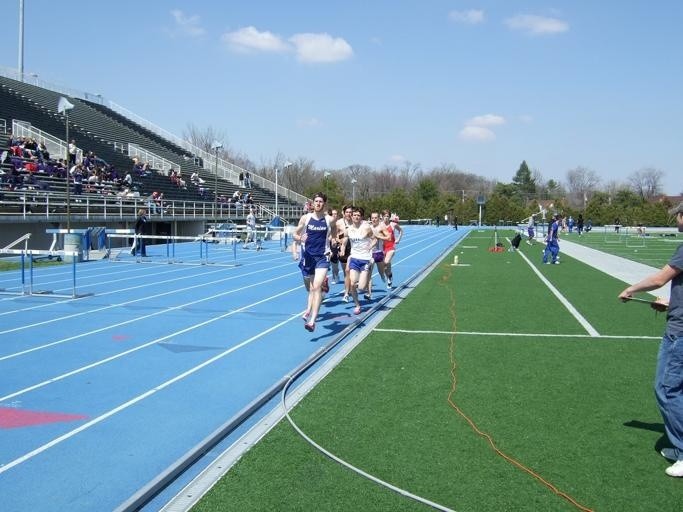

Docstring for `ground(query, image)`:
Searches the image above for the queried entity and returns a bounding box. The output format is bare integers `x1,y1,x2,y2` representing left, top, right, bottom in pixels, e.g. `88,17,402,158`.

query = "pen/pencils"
655,295,659,317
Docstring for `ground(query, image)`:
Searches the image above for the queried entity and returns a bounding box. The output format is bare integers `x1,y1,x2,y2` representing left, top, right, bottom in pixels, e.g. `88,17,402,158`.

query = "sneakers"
352,305,361,316
660,447,682,479
341,293,350,303
363,293,372,302
304,321,315,334
386,273,393,290
301,310,310,321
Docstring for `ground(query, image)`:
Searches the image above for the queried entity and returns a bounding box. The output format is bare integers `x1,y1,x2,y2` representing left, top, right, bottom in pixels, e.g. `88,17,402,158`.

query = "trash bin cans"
63,233,83,263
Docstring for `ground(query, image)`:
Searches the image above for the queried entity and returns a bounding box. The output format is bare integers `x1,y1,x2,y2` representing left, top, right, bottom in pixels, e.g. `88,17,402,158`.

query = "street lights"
207,139,221,242
323,170,329,213
54,98,78,297
350,178,357,210
273,160,292,216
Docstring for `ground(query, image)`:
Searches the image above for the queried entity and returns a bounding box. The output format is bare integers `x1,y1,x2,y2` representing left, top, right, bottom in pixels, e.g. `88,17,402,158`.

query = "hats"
668,201,683,215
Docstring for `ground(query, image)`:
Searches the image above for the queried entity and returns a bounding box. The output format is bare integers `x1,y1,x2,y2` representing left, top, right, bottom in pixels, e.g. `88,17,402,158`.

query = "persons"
453,215,458,231
243,208,260,249
131,208,148,257
435,215,441,227
1,128,258,215
506,211,593,265
618,203,683,478
614,217,621,234
444,213,449,225
292,191,403,332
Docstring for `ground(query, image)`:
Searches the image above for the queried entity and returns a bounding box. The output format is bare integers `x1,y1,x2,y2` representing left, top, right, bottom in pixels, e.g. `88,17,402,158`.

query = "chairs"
0,76,69,216
147,130,210,215
69,97,146,215
209,171,305,217
